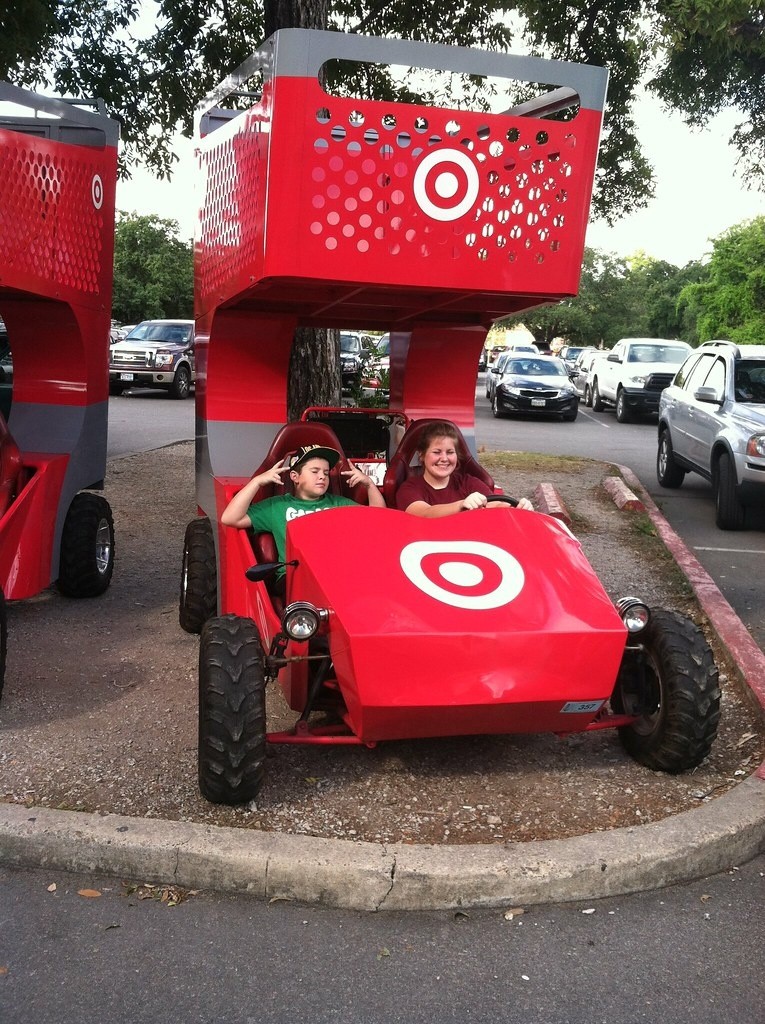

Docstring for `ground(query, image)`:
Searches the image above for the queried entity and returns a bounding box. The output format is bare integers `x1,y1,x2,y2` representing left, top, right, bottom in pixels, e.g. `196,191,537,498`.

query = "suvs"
654,338,765,530
591,338,696,422
108,319,195,400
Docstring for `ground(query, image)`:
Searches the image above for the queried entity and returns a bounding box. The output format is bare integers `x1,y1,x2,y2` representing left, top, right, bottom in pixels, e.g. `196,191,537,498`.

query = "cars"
109,320,148,345
336,329,392,395
479,340,613,408
176,407,722,806
489,353,581,422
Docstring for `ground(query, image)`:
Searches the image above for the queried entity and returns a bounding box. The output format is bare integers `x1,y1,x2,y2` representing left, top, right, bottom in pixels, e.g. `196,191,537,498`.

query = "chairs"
511,362,526,373
246,420,494,564
736,371,749,388
0,418,22,514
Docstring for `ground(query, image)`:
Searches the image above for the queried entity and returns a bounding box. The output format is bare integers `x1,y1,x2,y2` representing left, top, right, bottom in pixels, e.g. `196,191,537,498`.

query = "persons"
395,423,534,517
220,443,387,609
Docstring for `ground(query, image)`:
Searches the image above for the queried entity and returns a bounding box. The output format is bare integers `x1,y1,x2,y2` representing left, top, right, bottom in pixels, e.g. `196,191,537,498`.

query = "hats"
289,444,341,471
